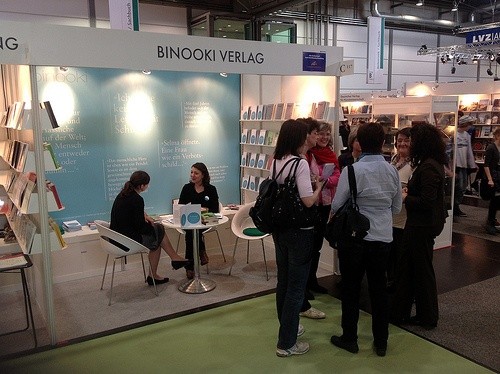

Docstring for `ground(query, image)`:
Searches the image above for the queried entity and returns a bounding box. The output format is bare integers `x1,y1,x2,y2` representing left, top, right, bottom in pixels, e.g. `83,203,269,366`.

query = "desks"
0,254,37,352
161,215,229,294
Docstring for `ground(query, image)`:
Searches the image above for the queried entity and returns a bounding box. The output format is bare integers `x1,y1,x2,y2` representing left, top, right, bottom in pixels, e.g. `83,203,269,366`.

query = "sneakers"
276,342,310,356
296,324,304,336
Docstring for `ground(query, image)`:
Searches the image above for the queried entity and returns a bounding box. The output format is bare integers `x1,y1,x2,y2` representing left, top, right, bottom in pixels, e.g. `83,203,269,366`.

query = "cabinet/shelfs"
51,205,247,278
0,122,67,256
240,118,326,194
459,110,500,208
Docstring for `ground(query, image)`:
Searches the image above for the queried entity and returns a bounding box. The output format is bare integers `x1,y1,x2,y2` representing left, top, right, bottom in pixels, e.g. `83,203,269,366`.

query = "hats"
459,115,474,124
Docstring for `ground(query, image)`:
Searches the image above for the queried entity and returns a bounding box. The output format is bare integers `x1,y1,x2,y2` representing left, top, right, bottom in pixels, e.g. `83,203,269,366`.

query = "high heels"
171,258,192,270
147,276,168,285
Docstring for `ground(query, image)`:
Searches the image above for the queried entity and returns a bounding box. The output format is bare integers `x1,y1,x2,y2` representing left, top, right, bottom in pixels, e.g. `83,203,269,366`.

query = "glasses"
190,174,200,178
314,132,320,136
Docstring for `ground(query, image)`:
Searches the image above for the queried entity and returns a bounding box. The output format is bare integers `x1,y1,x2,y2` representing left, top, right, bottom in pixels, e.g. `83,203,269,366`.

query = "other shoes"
495,221,500,227
187,271,193,279
200,253,209,266
458,211,467,217
331,335,359,353
306,291,315,300
313,285,329,294
298,307,326,319
373,340,387,357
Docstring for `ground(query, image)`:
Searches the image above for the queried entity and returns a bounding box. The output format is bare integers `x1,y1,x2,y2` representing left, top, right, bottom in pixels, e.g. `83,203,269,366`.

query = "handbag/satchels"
324,164,370,243
480,178,495,201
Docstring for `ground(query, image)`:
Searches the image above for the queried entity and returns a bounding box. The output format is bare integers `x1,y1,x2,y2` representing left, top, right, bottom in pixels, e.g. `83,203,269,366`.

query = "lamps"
438,6,458,18
220,72,227,78
60,66,68,71
441,54,500,81
432,84,440,91
390,0,423,14
142,70,151,75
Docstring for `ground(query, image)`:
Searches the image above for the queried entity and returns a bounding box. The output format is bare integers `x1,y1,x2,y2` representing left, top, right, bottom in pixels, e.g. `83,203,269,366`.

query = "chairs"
94,219,158,306
228,201,271,281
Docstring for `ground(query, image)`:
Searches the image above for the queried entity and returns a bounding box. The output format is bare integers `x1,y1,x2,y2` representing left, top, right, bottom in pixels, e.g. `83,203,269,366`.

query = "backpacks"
249,158,295,233
273,158,320,245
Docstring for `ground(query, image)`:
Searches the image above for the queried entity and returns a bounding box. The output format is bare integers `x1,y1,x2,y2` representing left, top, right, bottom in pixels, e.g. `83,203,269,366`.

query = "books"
42,142,60,169
201,212,219,226
0,101,37,269
240,101,327,192
62,220,82,233
87,222,97,230
45,180,62,208
48,215,66,248
39,101,59,129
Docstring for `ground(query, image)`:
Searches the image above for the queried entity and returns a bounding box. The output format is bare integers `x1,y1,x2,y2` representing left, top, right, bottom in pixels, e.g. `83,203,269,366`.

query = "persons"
109,171,193,286
177,162,219,280
330,122,403,357
484,128,500,233
296,111,478,331
269,119,327,357
